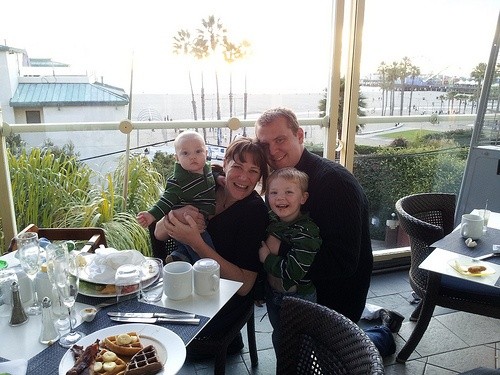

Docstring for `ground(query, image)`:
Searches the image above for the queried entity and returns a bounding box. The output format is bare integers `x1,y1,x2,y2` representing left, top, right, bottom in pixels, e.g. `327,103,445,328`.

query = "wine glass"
17,232,86,349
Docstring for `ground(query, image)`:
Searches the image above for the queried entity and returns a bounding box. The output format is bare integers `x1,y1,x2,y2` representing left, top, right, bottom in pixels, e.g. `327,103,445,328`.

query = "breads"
89,331,164,375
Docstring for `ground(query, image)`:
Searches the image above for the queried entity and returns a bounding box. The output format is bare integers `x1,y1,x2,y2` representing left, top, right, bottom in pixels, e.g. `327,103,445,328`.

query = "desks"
418,209,500,289
0,277,244,375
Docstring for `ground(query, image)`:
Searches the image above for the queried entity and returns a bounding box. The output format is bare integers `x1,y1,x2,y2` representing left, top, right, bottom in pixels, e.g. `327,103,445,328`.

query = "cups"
139,258,163,302
115,265,139,312
480,209,491,231
460,214,484,240
193,258,220,296
163,261,193,300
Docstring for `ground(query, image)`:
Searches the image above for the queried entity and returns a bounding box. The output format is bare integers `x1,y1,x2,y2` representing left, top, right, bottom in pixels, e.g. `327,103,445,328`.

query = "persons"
154,138,269,360
260,167,322,375
254,107,405,375
136,130,226,264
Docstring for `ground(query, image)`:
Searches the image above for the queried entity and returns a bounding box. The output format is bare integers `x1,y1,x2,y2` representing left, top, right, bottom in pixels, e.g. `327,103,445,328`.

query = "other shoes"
186,330,245,363
378,307,405,333
165,255,182,264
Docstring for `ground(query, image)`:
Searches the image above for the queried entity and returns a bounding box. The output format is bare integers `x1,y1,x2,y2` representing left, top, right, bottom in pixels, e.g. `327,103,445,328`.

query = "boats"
360,74,500,116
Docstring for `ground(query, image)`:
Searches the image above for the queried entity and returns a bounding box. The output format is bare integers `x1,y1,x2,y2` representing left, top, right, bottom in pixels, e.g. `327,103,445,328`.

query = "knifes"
107,312,200,325
472,252,500,261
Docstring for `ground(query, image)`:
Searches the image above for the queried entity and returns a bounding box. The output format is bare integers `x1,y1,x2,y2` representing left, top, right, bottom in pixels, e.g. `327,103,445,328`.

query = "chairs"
7,224,108,255
149,222,259,375
395,193,457,365
276,296,384,375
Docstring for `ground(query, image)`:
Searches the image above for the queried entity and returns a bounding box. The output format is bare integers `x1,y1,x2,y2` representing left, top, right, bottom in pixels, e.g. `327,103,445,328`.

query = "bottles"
8,281,61,344
36,263,52,301
40,297,59,344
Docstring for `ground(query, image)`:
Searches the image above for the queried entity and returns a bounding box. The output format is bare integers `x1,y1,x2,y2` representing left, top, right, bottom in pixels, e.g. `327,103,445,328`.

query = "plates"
69,254,159,297
58,322,187,375
